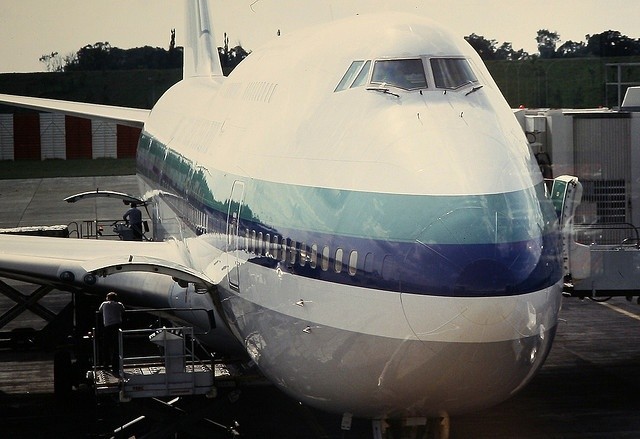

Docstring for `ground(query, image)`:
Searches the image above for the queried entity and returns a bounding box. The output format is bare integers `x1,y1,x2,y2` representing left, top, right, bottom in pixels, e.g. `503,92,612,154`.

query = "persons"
122,203,142,241
99,292,126,376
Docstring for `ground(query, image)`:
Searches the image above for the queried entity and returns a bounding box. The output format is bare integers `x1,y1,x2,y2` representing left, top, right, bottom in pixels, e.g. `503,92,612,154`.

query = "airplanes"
0,0,564,439
